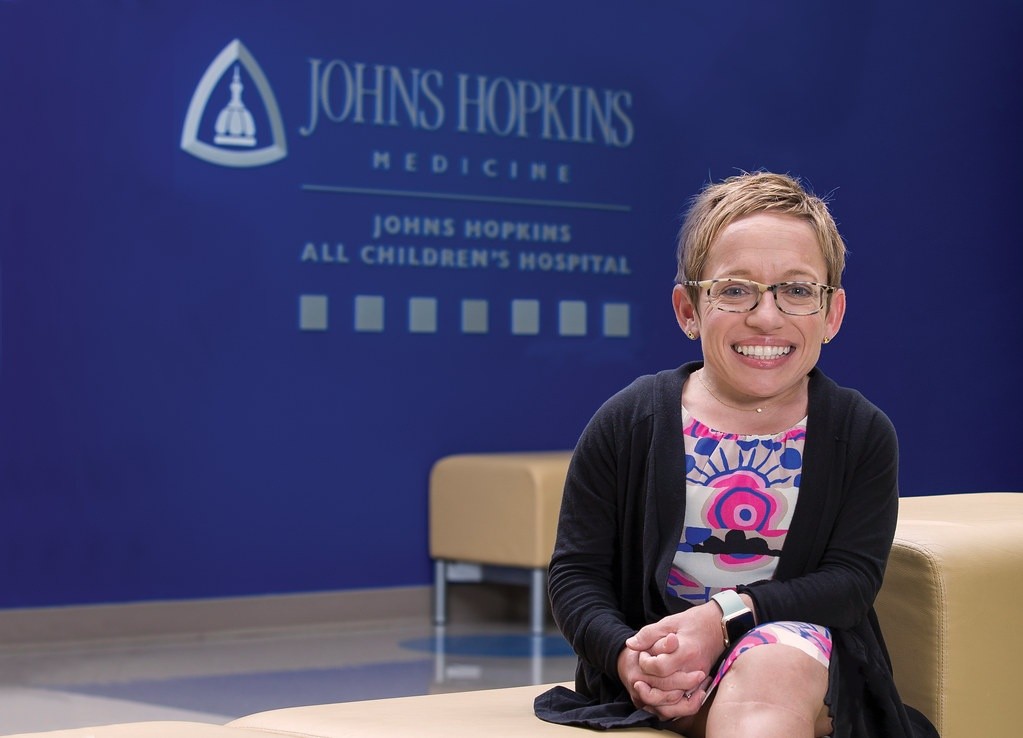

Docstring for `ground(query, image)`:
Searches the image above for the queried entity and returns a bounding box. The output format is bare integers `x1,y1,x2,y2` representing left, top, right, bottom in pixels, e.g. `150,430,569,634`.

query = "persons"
534,172,940,738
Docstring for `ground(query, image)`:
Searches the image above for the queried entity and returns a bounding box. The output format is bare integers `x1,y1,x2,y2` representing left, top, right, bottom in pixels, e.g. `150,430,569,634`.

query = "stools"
872,490,1023,738
429,447,584,638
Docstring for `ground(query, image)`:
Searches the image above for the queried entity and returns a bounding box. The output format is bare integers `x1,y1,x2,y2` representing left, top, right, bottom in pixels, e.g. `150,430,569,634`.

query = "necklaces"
695,370,808,413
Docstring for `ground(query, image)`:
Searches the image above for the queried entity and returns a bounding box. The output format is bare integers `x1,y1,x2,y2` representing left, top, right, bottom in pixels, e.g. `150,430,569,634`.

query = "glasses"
682,278,838,316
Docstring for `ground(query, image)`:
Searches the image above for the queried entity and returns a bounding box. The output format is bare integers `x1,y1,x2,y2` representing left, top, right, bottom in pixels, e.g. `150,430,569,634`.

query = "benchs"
0,680,690,738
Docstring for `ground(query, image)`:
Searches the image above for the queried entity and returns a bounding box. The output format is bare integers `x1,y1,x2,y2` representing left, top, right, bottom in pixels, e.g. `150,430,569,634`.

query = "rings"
683,693,691,699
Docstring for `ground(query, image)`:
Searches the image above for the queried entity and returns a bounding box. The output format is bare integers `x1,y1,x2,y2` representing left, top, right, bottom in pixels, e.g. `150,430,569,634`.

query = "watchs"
711,590,756,649
